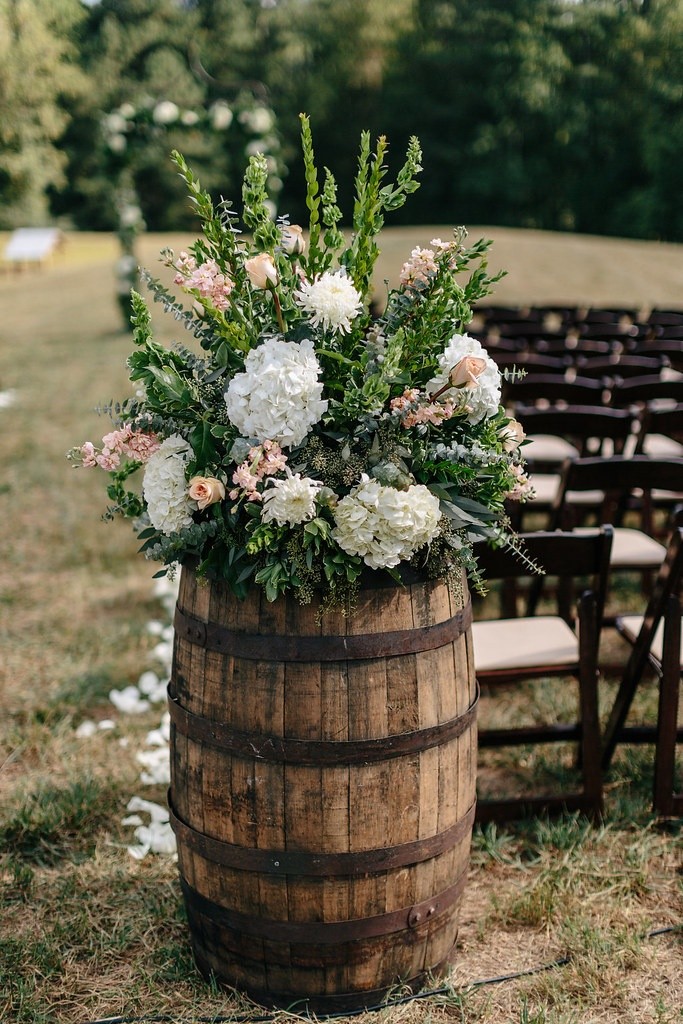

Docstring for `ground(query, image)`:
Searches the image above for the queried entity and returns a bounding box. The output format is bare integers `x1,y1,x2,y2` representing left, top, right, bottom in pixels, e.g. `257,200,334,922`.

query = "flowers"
63,108,546,622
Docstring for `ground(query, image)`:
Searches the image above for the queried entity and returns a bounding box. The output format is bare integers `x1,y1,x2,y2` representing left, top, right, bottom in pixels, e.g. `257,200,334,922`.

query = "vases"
170,567,475,1017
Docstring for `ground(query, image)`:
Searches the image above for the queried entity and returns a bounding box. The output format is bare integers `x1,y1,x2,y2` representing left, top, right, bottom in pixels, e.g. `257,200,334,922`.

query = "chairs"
449,302,683,834
